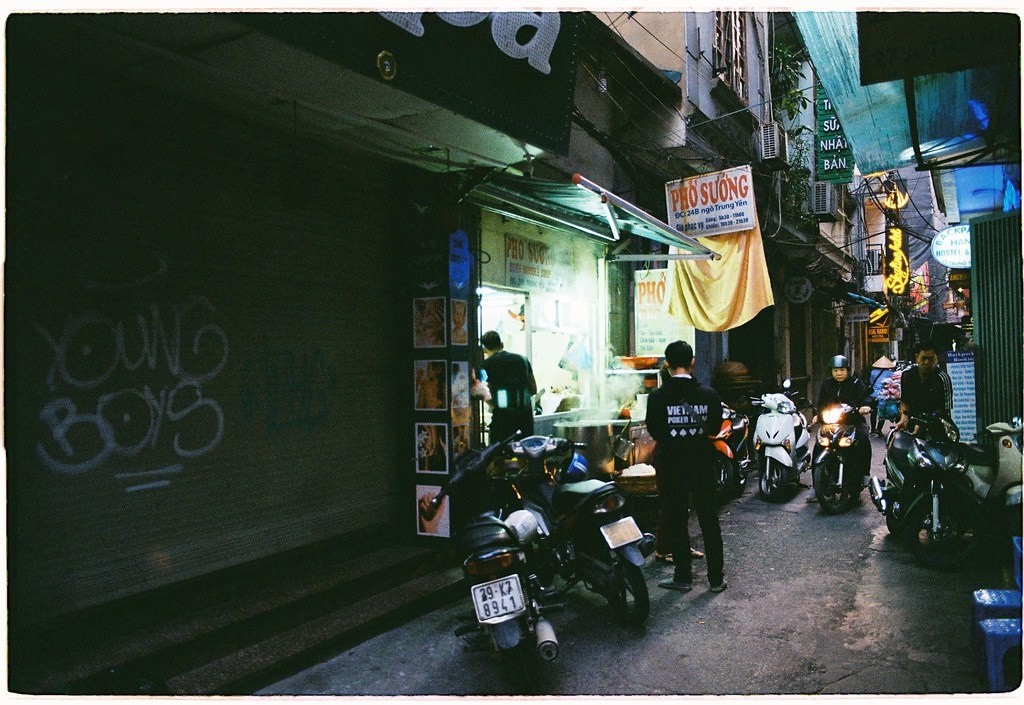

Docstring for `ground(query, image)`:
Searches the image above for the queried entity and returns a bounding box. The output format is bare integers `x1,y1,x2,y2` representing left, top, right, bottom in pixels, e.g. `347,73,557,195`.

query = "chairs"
972,536,1023,664
978,618,1022,691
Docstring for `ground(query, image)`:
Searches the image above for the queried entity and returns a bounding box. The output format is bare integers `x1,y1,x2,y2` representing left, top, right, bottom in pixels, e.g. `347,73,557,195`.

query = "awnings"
482,171,722,260
846,292,886,311
791,11,1022,224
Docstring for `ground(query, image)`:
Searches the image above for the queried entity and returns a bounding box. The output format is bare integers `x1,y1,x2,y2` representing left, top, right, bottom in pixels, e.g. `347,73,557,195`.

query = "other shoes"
871,430,877,435
876,431,884,436
711,579,727,592
657,577,692,590
690,547,704,559
805,489,817,500
655,553,673,563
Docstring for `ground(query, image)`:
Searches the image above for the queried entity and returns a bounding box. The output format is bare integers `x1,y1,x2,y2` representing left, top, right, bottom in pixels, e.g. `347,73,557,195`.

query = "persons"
869,355,896,437
450,302,466,342
896,344,953,541
805,355,873,502
645,341,729,593
415,299,444,345
481,332,536,475
417,365,446,409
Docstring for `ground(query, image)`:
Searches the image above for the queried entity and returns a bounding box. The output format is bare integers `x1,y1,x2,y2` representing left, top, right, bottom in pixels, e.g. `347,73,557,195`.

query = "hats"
871,355,894,368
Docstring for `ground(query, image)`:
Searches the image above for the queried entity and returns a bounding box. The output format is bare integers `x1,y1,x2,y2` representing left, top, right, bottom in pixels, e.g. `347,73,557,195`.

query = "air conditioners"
866,250,883,273
760,122,790,167
813,181,841,223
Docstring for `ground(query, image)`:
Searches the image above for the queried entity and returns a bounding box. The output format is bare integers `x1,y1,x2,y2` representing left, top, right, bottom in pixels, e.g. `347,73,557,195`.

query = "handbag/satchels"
868,386,874,394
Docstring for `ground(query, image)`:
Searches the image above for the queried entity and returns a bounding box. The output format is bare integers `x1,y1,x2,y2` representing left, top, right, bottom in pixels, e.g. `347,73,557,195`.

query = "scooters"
431,427,560,667
748,380,811,501
506,438,650,621
714,400,749,500
949,418,1023,564
871,410,966,556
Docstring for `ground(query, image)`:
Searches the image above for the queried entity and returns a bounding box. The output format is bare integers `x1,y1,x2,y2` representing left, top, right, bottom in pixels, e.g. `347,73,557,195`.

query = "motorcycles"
808,401,870,510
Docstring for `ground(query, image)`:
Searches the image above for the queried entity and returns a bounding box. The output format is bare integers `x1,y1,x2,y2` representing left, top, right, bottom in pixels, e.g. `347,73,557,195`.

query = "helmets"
554,451,588,484
503,508,543,546
828,355,850,367
